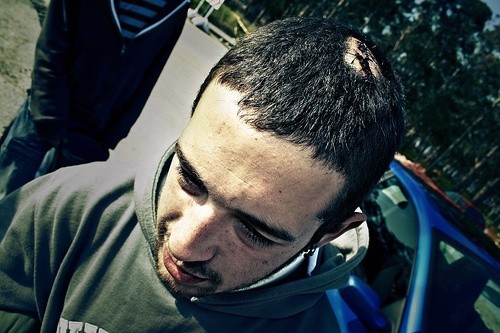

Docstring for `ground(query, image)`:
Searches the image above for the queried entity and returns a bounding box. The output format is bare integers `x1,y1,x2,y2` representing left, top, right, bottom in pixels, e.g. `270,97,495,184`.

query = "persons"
0,0,190,200
0,16,406,333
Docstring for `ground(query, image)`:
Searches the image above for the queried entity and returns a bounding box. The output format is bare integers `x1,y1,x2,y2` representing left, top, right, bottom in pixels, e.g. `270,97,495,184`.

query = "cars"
359,158,500,333
